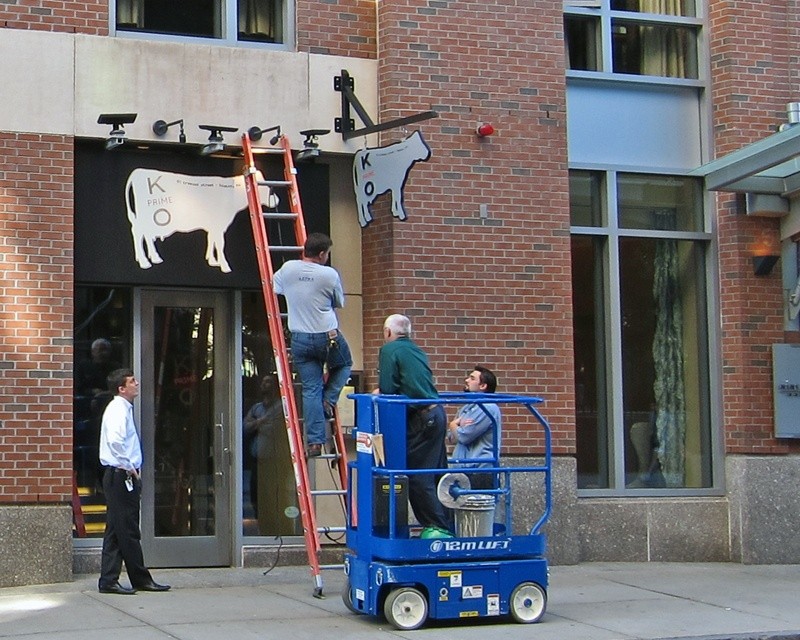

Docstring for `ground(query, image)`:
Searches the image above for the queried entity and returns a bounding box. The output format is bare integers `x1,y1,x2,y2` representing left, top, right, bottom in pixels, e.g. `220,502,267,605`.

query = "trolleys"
342,389,550,630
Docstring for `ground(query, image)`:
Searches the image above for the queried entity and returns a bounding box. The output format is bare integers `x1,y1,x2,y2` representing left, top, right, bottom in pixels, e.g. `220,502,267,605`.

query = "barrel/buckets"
454,494,496,539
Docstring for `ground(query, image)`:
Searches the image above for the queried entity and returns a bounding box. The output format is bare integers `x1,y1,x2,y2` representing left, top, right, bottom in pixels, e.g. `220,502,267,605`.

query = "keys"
128,480,132,486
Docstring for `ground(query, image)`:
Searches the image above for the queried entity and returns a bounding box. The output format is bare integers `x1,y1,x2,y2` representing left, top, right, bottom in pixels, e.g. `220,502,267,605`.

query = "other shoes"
309,444,322,456
323,402,334,418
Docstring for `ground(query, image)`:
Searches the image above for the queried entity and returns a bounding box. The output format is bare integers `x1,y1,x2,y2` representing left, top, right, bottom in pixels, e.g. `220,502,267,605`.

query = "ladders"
71,470,108,538
241,131,357,599
156,308,213,536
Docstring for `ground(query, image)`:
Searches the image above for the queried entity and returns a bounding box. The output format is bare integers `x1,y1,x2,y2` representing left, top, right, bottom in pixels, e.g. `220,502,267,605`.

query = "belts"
417,403,438,416
106,465,127,473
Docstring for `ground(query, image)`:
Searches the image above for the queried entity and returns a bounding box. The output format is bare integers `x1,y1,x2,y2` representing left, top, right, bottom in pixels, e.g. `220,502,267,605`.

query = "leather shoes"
133,579,171,591
99,582,136,594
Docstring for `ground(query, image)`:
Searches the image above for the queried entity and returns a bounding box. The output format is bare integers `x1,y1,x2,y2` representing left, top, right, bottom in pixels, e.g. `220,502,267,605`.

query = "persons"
273,232,353,459
98,367,172,595
79,337,297,536
624,402,658,489
370,314,502,532
